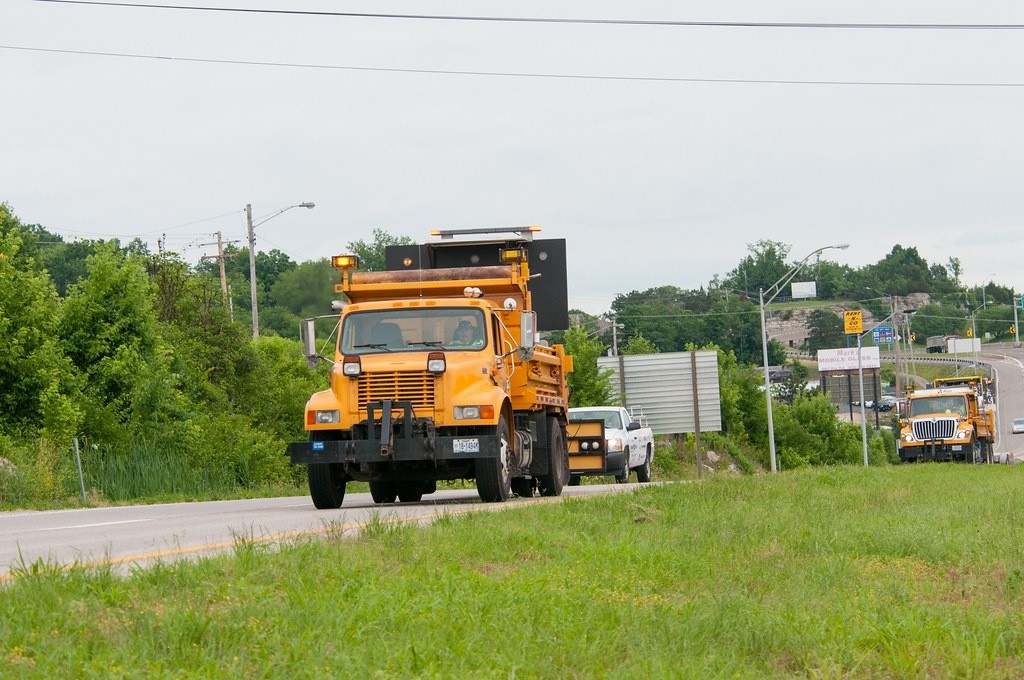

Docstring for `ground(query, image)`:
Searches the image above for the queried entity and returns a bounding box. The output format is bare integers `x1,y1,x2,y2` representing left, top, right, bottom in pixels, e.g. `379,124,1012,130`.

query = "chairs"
371,322,403,349
453,326,483,340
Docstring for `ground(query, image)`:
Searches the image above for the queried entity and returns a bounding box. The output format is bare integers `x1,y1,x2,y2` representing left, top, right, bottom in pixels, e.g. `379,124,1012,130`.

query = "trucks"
926,335,963,353
895,375,996,465
283,225,608,509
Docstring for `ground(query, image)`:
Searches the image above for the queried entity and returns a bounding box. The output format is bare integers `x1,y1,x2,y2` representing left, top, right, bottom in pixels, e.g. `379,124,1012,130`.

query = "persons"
950,398,966,415
447,320,483,346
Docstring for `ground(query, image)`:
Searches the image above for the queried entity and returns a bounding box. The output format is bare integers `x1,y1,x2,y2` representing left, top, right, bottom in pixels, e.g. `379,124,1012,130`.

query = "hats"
458,320,472,329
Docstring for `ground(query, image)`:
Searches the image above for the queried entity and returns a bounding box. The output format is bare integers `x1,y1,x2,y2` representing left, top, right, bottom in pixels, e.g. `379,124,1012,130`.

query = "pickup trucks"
568,407,654,486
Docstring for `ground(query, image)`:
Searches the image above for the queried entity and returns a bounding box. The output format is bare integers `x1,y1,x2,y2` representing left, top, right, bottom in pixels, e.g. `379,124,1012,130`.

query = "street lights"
246,202,315,341
982,273,996,310
759,243,852,472
866,287,902,371
857,309,919,466
972,301,993,376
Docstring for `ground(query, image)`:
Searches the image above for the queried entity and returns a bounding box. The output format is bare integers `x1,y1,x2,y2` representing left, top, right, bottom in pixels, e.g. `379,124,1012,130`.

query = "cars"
1011,417,1024,434
852,384,895,412
